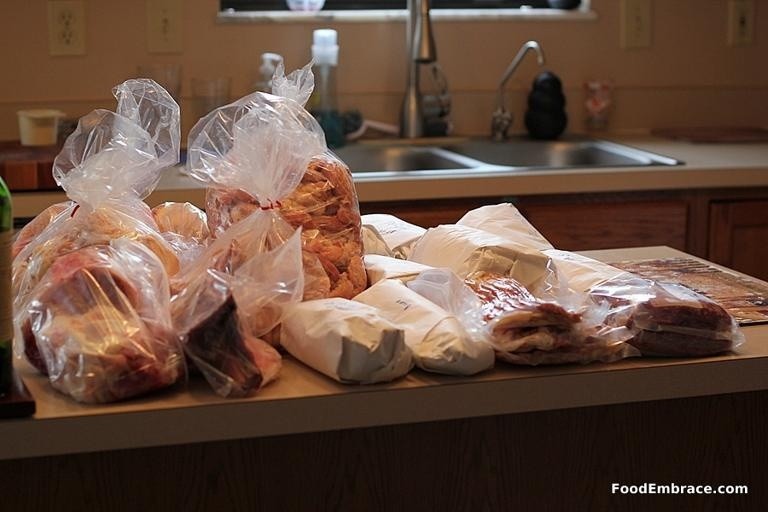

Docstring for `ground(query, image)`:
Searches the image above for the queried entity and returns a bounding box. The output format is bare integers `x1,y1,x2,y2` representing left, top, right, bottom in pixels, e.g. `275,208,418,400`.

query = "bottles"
307,31,347,146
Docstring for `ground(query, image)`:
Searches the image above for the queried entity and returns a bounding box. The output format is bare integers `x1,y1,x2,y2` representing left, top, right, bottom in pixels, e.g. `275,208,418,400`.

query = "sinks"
440,132,685,174
330,142,473,179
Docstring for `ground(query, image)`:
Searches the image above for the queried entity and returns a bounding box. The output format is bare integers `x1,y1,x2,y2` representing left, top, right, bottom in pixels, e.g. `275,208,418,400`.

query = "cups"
15,109,60,146
581,77,612,134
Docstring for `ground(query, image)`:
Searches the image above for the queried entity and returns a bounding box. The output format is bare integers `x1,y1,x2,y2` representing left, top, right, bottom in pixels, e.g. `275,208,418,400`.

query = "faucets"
493,40,544,140
400,0,436,140
